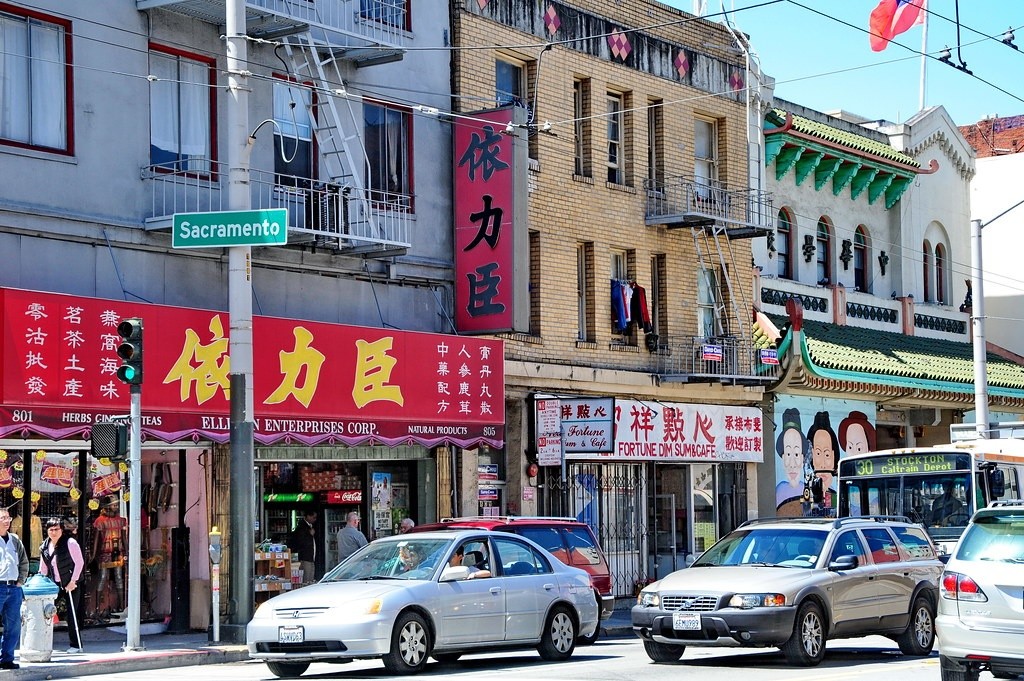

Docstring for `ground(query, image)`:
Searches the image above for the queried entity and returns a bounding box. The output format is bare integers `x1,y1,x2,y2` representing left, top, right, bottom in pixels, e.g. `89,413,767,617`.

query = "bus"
810,439,1024,565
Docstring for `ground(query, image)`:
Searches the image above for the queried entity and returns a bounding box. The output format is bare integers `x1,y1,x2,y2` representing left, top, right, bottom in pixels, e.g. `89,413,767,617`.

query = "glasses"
0,517,13,522
50,528,61,532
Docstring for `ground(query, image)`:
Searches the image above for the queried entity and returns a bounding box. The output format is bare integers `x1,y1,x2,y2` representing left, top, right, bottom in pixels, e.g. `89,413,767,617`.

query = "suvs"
631,513,944,666
399,515,614,621
934,499,1024,681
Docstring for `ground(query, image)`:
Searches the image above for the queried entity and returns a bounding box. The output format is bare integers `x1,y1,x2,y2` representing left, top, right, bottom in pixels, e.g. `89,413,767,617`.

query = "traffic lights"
117,317,142,385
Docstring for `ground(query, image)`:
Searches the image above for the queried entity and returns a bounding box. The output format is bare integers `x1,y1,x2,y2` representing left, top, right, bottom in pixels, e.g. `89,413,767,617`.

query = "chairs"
505,562,537,575
790,540,816,561
752,538,778,563
465,551,488,570
860,538,885,558
838,534,854,555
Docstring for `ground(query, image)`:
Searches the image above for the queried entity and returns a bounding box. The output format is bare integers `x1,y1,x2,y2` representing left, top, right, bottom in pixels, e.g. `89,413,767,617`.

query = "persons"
433,545,491,580
38,516,85,654
400,518,415,533
298,506,318,582
0,507,29,670
90,493,132,619
931,479,959,524
390,542,426,579
10,493,43,575
337,512,370,566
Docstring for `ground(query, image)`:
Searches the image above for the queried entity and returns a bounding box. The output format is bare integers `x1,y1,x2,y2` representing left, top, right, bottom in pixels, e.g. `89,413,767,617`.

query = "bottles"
329,510,350,551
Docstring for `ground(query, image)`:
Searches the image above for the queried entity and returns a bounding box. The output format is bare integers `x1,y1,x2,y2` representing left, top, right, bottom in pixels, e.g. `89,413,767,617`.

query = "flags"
869,0,925,52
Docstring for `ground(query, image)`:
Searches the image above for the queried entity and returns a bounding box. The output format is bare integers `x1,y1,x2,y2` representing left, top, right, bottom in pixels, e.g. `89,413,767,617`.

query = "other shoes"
0,660,19,669
67,647,84,653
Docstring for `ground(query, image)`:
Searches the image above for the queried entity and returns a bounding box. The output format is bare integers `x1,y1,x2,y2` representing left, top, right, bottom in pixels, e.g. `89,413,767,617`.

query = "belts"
0,580,16,586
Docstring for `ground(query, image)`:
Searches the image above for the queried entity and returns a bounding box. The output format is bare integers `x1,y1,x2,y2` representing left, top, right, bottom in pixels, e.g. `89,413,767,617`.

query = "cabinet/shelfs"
255,548,293,610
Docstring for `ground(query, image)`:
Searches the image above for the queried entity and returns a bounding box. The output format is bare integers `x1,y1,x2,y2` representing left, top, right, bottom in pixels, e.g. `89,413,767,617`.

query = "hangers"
610,277,635,285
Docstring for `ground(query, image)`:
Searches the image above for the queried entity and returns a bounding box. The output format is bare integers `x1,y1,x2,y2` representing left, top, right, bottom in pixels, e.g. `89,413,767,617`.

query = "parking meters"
209,526,225,646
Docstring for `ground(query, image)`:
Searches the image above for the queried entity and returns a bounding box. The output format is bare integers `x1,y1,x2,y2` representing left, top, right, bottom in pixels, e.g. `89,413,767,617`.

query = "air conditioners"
308,192,351,235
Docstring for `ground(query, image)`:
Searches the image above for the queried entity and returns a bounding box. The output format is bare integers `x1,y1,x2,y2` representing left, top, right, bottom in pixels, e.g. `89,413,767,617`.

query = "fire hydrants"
18,571,60,664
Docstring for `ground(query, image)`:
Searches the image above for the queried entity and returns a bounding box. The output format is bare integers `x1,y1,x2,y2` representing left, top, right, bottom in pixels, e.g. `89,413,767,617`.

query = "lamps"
838,282,844,287
907,294,914,298
844,286,860,291
755,266,763,272
878,406,885,412
820,278,828,285
891,291,896,300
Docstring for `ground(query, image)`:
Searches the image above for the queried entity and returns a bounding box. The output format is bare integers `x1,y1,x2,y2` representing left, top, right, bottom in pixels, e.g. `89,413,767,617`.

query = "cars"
246,531,599,678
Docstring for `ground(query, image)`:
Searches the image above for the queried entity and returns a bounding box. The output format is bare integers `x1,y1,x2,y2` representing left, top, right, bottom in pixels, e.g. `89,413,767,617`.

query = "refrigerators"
264,493,314,545
316,489,361,580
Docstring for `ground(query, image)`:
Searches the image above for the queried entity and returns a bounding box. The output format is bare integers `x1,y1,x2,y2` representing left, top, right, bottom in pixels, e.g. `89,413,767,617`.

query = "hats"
102,494,120,507
